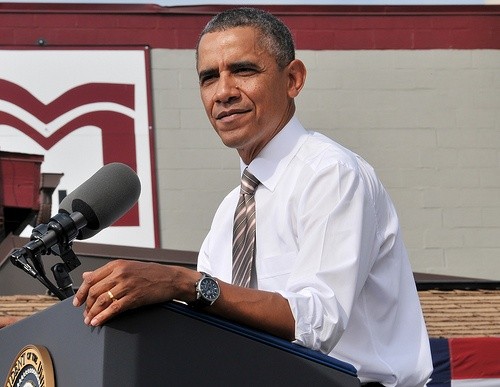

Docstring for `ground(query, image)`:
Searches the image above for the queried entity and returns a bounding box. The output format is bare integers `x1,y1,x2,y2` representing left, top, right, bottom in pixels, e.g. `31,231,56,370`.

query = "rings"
108,290,117,303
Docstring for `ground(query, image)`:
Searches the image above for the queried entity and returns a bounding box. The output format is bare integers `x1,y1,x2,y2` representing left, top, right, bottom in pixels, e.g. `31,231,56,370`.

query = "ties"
232,165,259,289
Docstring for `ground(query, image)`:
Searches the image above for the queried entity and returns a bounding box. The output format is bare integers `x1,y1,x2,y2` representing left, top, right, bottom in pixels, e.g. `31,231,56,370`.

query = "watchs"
186,272,220,309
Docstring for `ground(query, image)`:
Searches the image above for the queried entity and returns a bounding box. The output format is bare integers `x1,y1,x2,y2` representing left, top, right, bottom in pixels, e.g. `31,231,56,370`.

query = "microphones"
30,162,142,243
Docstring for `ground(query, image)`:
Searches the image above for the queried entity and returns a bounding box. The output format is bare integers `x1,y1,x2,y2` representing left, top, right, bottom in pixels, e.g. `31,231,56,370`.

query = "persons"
73,8,434,387
